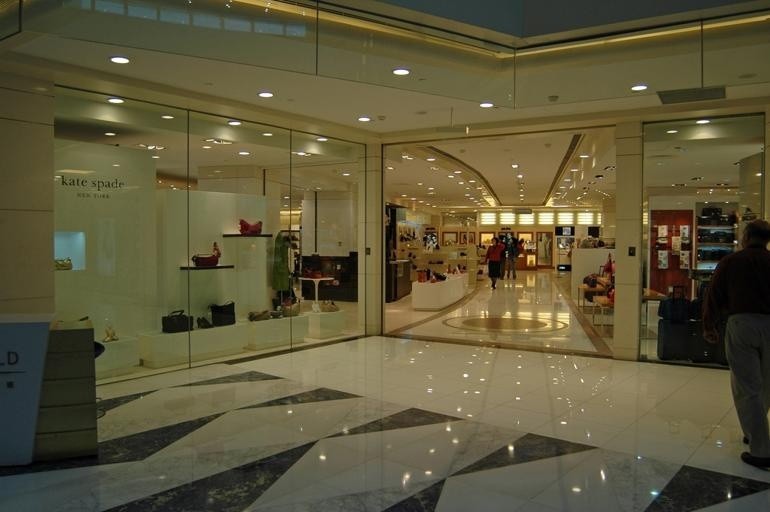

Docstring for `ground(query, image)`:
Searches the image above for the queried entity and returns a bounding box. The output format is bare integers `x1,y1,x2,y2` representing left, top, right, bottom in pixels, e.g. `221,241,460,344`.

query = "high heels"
197,317,213,329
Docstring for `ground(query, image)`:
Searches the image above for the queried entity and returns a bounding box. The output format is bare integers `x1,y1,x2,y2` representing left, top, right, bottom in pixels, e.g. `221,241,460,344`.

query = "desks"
298,277,335,303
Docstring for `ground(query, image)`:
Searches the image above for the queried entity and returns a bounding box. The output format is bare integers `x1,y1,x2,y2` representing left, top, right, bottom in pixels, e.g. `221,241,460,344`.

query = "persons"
699,218,769,470
498,236,508,280
431,235,439,249
504,238,520,280
426,233,433,249
517,239,526,250
282,236,297,298
484,237,504,289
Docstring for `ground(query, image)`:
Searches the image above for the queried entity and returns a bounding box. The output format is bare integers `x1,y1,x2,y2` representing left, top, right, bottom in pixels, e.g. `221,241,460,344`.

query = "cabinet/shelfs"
389,243,481,311
576,195,739,326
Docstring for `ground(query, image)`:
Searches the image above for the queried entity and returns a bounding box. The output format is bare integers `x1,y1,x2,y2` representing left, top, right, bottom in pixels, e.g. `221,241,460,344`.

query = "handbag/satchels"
210,302,235,326
658,298,703,320
192,255,218,266
283,303,300,316
162,310,193,333
240,219,262,234
249,311,270,321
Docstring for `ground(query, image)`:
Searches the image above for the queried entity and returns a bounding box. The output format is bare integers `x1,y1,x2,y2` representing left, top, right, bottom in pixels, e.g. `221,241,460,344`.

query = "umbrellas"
269,231,290,305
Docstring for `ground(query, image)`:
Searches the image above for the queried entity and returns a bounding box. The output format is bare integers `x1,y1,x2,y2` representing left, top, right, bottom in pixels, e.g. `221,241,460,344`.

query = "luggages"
657,285,725,363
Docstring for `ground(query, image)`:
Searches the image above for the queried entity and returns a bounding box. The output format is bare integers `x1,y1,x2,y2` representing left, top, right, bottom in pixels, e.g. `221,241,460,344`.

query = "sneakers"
741,435,770,471
